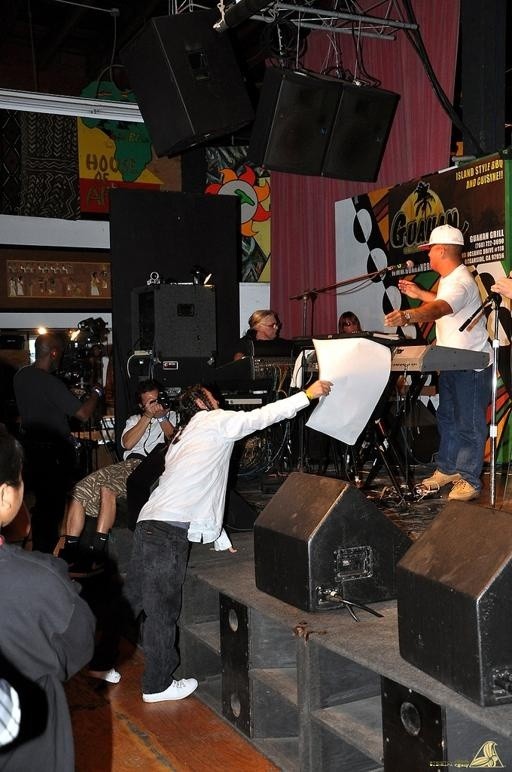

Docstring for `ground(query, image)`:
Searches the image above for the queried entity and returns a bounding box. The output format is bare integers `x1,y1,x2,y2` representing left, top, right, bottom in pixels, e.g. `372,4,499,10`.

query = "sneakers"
84,666,120,683
449,479,481,501
144,677,198,703
59,548,108,577
422,470,460,489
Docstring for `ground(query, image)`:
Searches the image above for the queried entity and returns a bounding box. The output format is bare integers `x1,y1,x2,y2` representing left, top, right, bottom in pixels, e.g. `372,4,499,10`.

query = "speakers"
119,11,257,159
253,472,413,613
388,400,438,465
396,500,511,706
138,285,217,391
248,66,343,176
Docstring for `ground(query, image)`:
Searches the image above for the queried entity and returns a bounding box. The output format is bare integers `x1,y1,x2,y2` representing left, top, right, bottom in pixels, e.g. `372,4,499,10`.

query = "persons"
88,380,334,703
339,312,361,335
13,333,104,555
490,271,512,300
232,310,280,360
384,224,494,502
0,430,97,772
60,379,180,578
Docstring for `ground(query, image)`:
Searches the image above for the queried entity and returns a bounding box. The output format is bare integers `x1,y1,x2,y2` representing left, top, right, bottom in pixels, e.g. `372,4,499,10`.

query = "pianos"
306,346,490,374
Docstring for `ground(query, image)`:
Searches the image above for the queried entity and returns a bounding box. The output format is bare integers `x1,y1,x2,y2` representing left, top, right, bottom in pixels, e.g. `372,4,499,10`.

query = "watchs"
404,310,411,324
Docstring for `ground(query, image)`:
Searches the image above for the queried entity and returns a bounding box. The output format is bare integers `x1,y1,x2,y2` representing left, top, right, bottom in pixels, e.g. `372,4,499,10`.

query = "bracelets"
93,389,102,397
158,415,167,423
305,390,313,401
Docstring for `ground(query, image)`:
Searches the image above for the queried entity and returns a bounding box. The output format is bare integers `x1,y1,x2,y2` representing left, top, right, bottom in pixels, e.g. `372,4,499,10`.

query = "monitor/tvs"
321,81,402,183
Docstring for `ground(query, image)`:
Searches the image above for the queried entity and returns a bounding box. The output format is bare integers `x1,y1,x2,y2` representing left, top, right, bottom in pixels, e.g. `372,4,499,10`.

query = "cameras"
150,391,170,409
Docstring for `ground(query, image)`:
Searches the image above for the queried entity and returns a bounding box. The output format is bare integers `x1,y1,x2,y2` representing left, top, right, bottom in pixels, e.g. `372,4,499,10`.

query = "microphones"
379,259,414,276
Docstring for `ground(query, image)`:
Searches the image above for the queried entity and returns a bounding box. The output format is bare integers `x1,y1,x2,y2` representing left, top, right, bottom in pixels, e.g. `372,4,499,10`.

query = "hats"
418,225,466,249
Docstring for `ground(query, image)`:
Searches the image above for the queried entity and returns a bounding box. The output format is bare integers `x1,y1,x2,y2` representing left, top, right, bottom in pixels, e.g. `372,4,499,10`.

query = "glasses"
264,322,277,330
344,320,357,326
